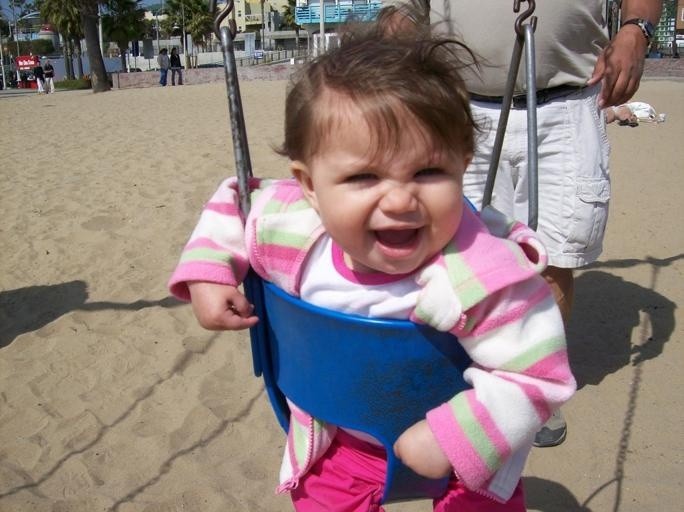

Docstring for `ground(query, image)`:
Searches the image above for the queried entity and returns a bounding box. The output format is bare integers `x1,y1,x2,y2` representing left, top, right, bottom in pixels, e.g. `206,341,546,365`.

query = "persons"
156,48,170,87
166,1,577,512
42,59,55,94
379,1,664,447
34,61,45,96
169,47,184,86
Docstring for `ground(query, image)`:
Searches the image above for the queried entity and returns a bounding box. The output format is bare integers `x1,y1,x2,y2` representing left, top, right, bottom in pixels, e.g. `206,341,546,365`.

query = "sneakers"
532,404,568,448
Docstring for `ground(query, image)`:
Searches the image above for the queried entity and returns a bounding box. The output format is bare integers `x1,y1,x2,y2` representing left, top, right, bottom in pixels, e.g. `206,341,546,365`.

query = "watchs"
621,17,656,47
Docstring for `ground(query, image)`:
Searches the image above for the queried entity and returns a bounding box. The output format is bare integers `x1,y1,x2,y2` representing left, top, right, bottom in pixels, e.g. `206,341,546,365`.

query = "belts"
460,82,592,111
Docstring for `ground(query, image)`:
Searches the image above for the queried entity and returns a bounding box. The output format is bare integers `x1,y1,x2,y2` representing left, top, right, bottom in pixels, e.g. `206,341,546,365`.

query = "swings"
212,1,539,506
